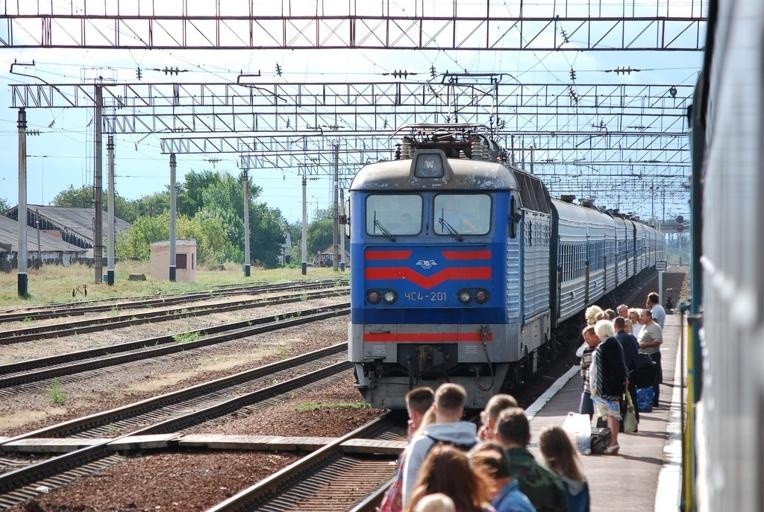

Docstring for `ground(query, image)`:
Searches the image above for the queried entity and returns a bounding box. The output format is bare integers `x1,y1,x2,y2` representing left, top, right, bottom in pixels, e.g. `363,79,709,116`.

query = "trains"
348,123,666,414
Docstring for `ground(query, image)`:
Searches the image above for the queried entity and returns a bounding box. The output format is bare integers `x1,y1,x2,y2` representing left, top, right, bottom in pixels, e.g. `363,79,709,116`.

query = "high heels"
603,444,619,455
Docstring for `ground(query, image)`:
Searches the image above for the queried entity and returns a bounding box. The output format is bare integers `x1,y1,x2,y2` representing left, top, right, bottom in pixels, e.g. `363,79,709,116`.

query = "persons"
579,292,666,455
399,383,590,512
440,201,477,232
392,213,416,235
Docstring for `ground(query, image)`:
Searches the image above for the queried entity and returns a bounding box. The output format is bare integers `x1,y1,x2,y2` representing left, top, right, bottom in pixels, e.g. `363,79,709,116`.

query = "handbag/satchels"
623,411,637,433
591,428,611,454
636,386,655,412
632,352,657,388
